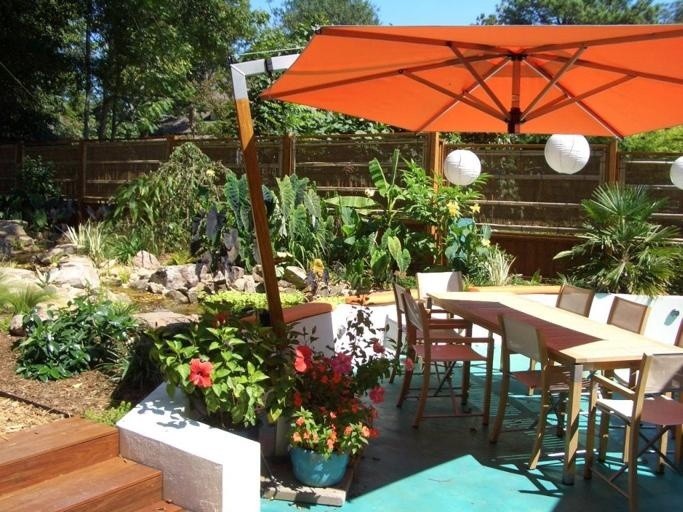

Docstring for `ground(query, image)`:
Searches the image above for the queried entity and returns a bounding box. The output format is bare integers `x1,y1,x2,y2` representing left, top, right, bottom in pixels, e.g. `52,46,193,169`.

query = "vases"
292,445,350,488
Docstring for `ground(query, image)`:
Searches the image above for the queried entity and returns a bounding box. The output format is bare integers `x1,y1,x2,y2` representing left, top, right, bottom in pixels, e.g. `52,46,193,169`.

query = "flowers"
143,293,415,456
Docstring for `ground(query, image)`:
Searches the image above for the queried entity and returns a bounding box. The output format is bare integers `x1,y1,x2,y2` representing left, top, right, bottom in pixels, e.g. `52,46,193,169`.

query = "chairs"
510,293,653,439
389,281,465,387
523,280,597,396
583,350,682,510
395,295,497,432
416,270,469,371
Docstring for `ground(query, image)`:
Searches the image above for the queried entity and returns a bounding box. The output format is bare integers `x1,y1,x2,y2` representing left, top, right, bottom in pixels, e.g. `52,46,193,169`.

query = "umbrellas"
224,22,682,352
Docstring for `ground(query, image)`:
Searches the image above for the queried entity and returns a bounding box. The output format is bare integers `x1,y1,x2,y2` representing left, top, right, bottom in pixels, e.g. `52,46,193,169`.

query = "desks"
427,290,682,488
